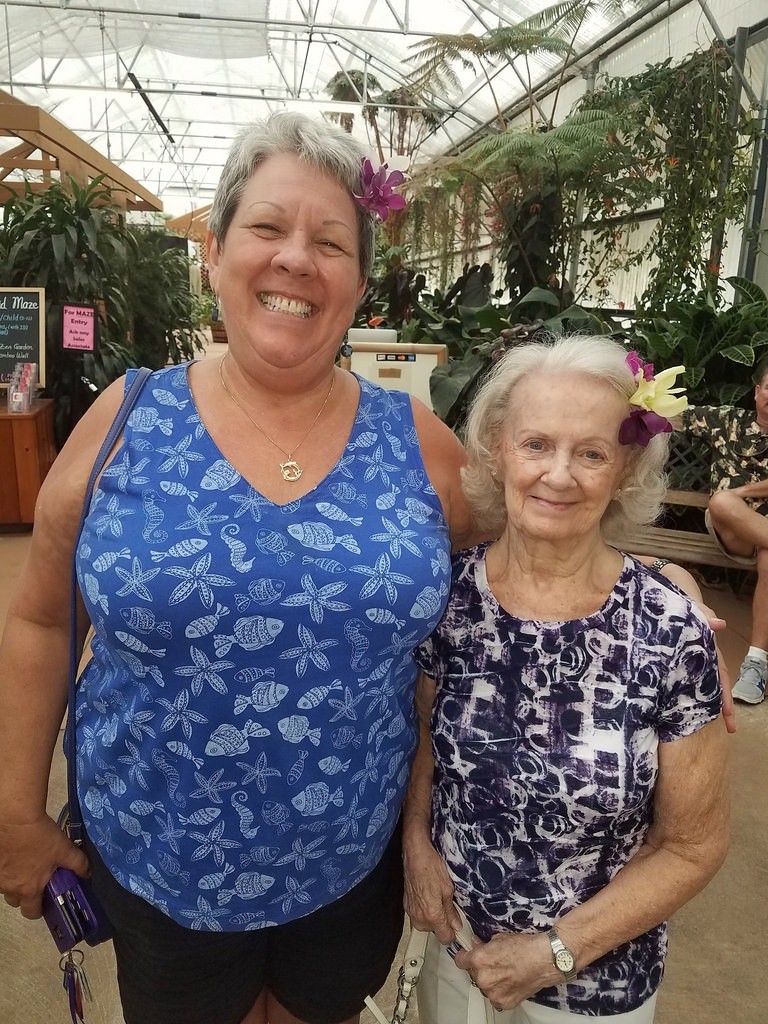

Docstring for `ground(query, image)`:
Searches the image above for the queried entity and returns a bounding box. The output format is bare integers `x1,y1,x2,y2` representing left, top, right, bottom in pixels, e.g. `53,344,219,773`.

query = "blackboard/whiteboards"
0,287,46,390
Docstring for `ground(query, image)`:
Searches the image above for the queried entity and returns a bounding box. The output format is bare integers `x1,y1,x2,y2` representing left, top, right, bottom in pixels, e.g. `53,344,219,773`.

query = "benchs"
615,489,757,571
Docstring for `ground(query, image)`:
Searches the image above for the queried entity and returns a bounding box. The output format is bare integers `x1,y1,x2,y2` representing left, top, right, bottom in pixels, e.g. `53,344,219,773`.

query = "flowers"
618,350,688,446
352,155,412,224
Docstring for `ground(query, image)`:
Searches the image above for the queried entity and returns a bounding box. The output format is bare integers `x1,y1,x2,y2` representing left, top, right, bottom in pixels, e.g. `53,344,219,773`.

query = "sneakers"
732,656,768,704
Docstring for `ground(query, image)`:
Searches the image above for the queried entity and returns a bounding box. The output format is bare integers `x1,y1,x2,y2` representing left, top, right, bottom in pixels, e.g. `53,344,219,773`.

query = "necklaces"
218,349,339,482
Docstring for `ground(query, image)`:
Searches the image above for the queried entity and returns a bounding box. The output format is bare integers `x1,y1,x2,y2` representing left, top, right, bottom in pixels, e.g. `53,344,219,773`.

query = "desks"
0,398,54,532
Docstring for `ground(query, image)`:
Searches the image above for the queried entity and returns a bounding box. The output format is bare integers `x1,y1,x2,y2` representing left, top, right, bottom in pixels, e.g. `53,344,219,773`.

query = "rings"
470,978,504,1012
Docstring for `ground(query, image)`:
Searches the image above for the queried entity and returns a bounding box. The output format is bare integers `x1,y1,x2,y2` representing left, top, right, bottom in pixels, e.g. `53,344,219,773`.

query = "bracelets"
648,557,674,577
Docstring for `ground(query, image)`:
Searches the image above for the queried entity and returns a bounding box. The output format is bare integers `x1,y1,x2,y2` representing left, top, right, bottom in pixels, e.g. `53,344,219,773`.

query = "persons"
0,113,737,1024
400,336,732,1024
671,354,768,705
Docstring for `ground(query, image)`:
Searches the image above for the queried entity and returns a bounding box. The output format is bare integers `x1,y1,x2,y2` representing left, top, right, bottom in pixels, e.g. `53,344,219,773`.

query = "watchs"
544,927,578,985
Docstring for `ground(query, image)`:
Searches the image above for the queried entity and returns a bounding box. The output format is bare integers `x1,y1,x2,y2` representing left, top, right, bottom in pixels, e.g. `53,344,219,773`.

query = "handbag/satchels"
57,801,116,947
